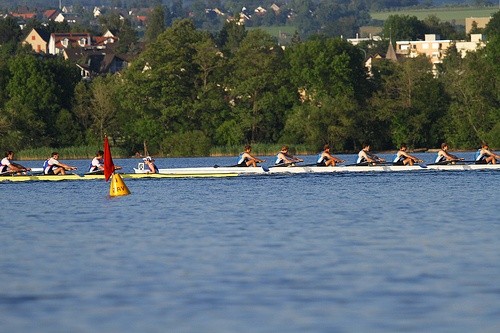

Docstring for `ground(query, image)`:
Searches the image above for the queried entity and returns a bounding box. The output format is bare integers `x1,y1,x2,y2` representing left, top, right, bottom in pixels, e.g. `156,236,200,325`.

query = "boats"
0,163,500,183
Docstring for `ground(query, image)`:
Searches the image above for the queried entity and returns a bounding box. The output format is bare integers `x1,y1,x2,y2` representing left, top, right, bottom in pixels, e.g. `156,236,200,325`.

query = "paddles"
33,168,77,175
303,160,344,166
418,160,449,169
71,168,102,177
261,162,285,172
0,170,29,174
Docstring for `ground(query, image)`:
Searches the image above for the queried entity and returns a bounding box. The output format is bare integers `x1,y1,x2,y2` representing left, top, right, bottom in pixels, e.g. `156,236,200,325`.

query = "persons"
316,144,344,166
143,156,159,173
356,143,385,166
275,145,300,167
475,145,500,164
237,145,264,167
44,152,77,176
435,143,458,164
88,151,104,172
392,144,418,166
0,151,27,175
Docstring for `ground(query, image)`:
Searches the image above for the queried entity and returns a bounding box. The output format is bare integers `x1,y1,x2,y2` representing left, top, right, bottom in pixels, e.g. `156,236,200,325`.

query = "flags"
104,137,115,181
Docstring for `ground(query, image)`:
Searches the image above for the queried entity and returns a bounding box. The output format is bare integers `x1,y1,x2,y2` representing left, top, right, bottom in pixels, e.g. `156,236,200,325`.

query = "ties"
142,156,152,162
